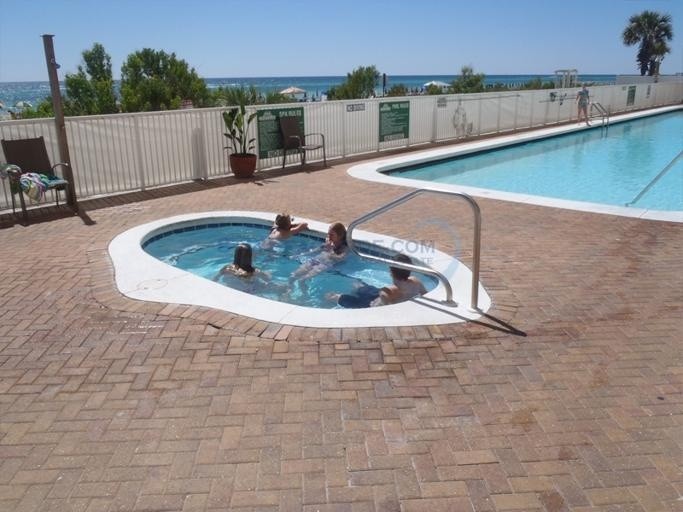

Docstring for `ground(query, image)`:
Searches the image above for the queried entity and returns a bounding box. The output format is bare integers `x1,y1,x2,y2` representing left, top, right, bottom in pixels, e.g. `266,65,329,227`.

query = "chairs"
275,116,326,171
0,135,78,226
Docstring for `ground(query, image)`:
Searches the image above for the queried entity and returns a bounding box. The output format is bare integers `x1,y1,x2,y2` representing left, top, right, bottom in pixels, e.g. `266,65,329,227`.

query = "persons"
319,253,428,309
289,222,351,296
259,211,310,255
212,241,287,297
574,81,594,126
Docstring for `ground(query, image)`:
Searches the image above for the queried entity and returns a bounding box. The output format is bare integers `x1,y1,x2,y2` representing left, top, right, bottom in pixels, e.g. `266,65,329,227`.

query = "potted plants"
220,101,260,178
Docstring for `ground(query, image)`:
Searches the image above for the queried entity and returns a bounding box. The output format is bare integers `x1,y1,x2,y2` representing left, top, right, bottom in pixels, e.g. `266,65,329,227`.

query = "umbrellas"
13,100,33,116
278,85,306,102
422,79,452,94
